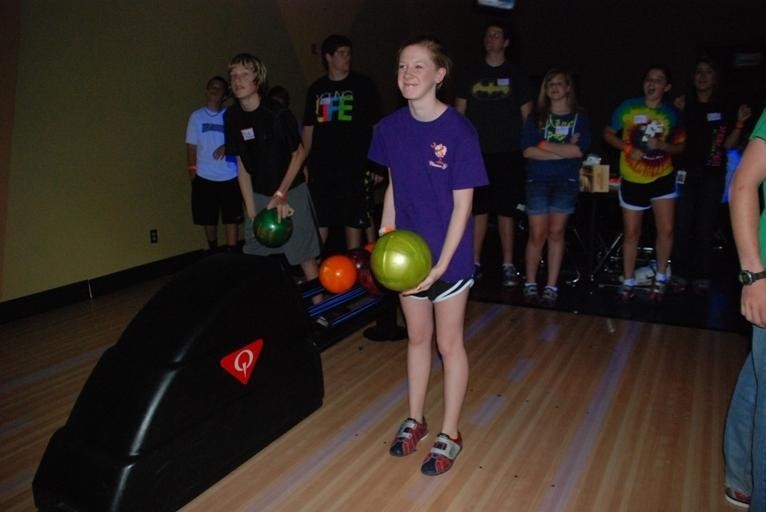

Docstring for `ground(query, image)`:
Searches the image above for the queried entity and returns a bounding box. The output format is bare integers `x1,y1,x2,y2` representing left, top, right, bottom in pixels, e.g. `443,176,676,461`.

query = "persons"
724,105,765,511
185,35,403,299
365,39,491,476
453,24,764,301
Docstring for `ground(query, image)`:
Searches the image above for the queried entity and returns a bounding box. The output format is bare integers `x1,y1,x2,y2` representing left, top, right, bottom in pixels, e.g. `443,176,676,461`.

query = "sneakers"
538,285,560,309
524,281,540,305
648,277,669,303
614,284,638,305
671,276,714,299
724,486,753,508
388,414,431,457
420,430,464,476
473,262,484,279
493,261,521,288
